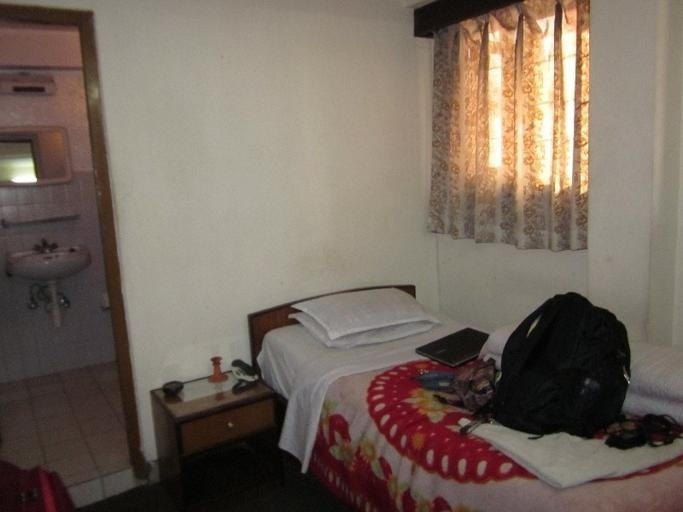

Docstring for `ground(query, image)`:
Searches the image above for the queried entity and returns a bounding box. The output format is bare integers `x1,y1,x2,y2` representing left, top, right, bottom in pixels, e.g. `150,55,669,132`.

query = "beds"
247,285,682,509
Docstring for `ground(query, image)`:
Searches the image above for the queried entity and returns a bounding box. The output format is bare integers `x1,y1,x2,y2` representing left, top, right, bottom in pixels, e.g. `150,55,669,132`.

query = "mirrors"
0,125,73,187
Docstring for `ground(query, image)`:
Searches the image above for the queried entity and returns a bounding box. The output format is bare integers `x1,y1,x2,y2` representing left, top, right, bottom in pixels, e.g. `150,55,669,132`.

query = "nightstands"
150,370,287,510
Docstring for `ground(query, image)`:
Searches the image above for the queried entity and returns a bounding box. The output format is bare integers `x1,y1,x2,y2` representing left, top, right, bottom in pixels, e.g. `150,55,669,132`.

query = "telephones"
231,359,259,382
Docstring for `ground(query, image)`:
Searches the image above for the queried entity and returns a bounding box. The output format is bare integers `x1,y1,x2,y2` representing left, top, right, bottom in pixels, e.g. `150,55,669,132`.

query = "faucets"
33,237,56,253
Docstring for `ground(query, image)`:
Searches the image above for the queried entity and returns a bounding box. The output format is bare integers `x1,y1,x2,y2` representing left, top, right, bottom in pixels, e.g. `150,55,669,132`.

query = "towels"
457,416,682,490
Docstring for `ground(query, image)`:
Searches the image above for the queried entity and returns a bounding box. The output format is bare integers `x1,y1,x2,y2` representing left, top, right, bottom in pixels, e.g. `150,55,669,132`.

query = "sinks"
6,244,89,282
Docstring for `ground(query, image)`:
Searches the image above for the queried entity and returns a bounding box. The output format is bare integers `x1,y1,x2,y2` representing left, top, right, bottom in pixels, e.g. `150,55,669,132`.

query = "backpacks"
492,293,630,438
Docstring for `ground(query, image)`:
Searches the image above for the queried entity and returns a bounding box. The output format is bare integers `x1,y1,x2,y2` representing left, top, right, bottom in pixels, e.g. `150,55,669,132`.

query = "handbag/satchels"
0,460,77,511
451,358,497,411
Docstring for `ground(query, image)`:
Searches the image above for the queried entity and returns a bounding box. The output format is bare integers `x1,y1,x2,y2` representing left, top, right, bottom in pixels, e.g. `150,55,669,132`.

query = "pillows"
290,287,444,341
285,313,435,349
